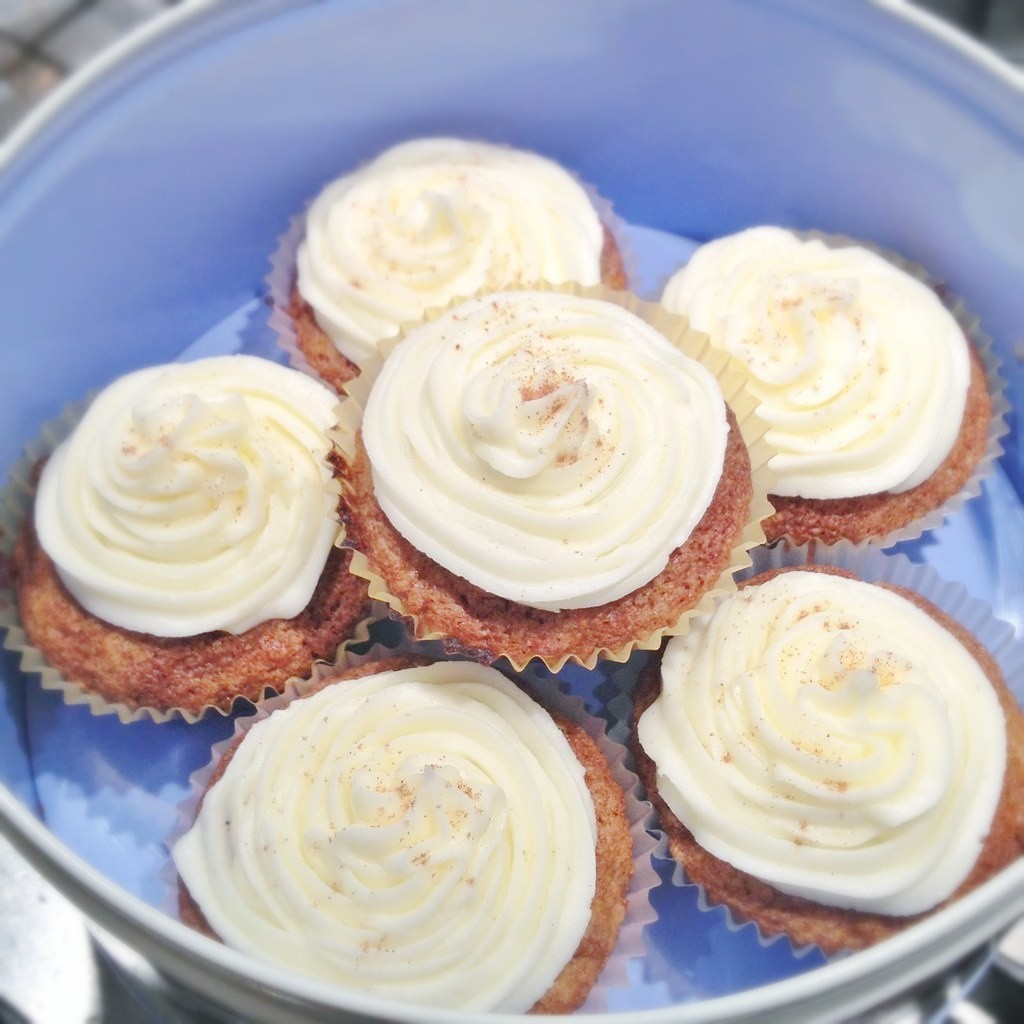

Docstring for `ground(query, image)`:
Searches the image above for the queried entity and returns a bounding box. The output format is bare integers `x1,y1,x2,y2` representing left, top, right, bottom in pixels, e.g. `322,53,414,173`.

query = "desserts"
18,135,1023,1009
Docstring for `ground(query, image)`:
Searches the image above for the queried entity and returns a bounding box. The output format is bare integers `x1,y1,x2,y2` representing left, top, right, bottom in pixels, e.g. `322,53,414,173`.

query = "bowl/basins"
0,1,1024,1024
